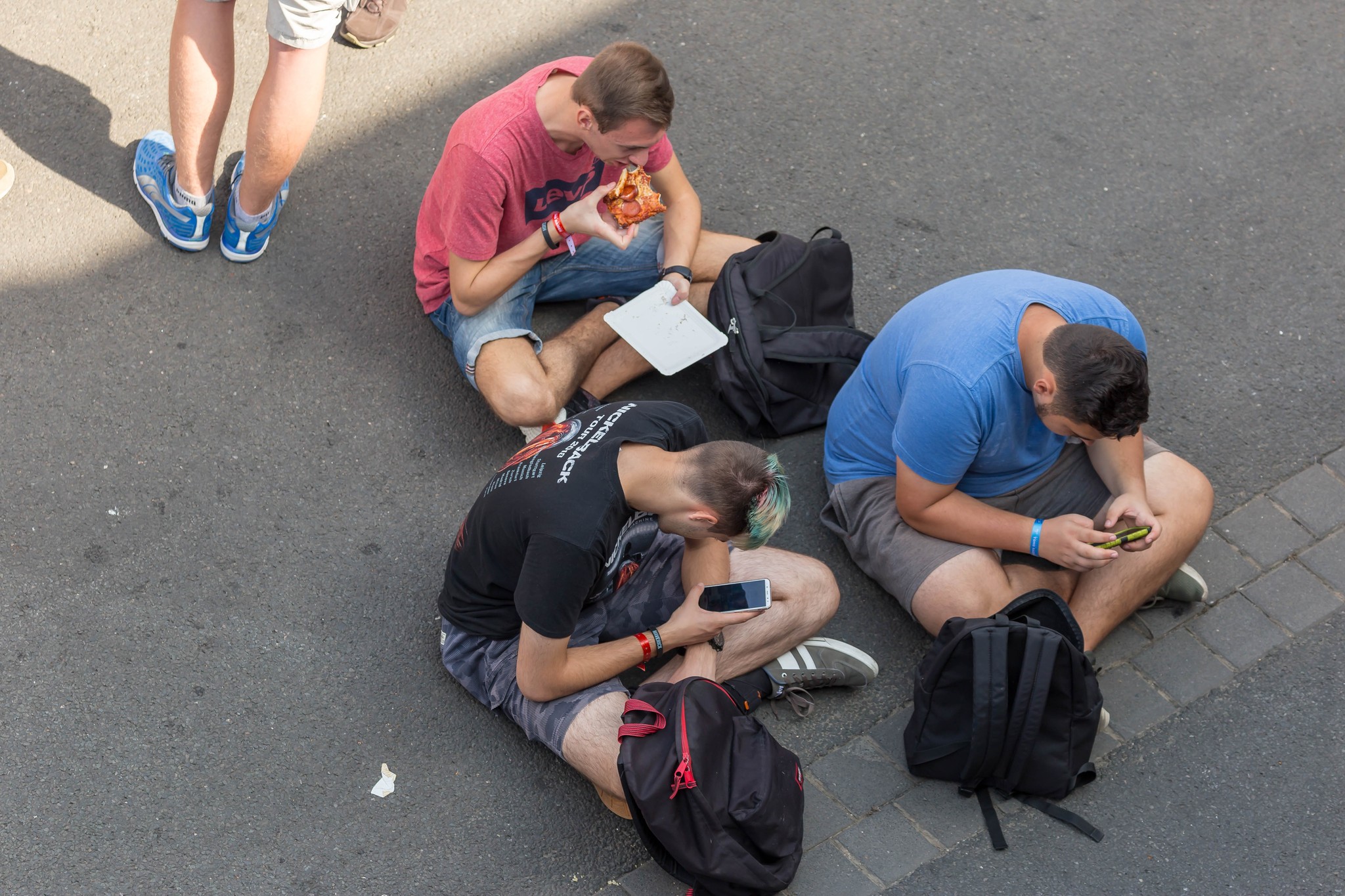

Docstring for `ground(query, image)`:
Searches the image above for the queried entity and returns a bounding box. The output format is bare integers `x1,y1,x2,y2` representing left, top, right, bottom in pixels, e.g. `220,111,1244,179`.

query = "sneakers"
133,129,213,253
219,152,289,264
759,635,881,720
1131,558,1209,637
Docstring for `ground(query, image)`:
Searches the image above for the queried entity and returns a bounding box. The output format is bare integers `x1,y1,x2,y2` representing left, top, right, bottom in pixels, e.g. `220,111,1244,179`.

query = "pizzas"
603,165,667,229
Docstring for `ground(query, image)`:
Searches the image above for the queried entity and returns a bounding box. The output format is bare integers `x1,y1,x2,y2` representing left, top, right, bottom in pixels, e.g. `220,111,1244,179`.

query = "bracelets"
541,220,560,250
552,212,576,257
631,633,651,671
646,626,663,665
1030,518,1045,556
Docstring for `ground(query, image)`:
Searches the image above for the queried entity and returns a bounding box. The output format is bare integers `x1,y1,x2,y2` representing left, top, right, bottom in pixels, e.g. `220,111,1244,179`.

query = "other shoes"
340,0,405,47
515,383,601,445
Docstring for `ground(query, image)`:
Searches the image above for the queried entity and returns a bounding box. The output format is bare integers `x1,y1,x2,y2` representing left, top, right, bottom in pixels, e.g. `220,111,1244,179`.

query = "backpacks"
902,588,1105,847
617,681,803,896
705,229,878,441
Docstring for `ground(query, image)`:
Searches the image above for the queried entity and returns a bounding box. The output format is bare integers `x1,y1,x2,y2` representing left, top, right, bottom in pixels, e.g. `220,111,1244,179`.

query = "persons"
131,0,409,262
437,399,880,823
412,39,760,445
817,269,1216,732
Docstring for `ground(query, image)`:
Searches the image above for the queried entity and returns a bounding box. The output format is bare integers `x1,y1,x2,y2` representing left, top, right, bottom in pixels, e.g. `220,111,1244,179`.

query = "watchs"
658,265,692,284
707,629,725,653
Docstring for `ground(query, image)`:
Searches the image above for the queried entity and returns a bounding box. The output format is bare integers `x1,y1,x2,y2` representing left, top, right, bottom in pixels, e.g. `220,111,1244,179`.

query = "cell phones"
699,579,772,613
1087,526,1151,549
